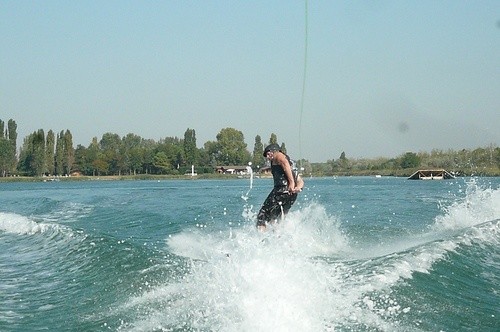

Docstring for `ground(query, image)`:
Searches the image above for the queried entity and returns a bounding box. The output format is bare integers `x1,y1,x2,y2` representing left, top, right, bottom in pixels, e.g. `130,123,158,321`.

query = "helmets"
263,144,281,157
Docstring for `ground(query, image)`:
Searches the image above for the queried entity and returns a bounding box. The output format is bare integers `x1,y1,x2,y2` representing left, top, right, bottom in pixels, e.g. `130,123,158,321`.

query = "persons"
254,143,305,230
